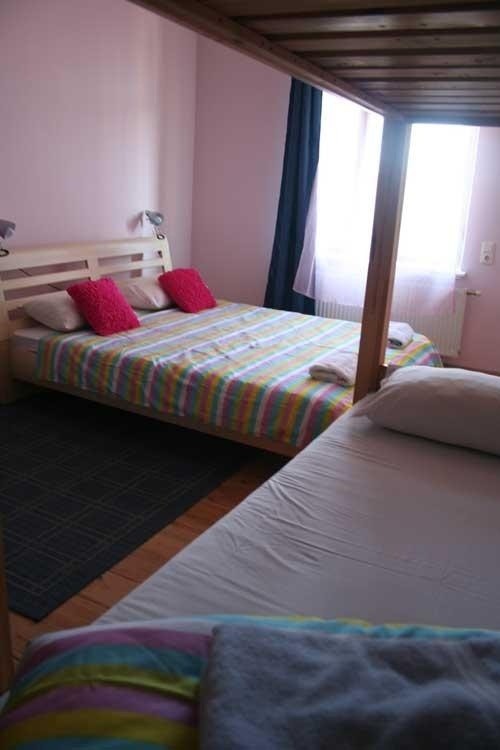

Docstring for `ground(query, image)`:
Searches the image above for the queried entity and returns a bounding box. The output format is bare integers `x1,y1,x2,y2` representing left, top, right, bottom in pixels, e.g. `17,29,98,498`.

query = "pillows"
354,365,499,458
20,267,218,337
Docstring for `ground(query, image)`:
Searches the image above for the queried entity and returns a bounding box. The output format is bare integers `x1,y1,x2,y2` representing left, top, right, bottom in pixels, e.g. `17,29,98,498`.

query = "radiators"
315,287,467,360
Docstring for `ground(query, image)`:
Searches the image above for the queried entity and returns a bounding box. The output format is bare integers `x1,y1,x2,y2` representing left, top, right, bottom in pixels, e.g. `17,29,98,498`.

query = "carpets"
1,391,250,626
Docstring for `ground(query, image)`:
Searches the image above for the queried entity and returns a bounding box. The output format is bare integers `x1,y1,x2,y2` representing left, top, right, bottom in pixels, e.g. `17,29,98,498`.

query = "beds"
0,380,499,749
0,234,439,461
